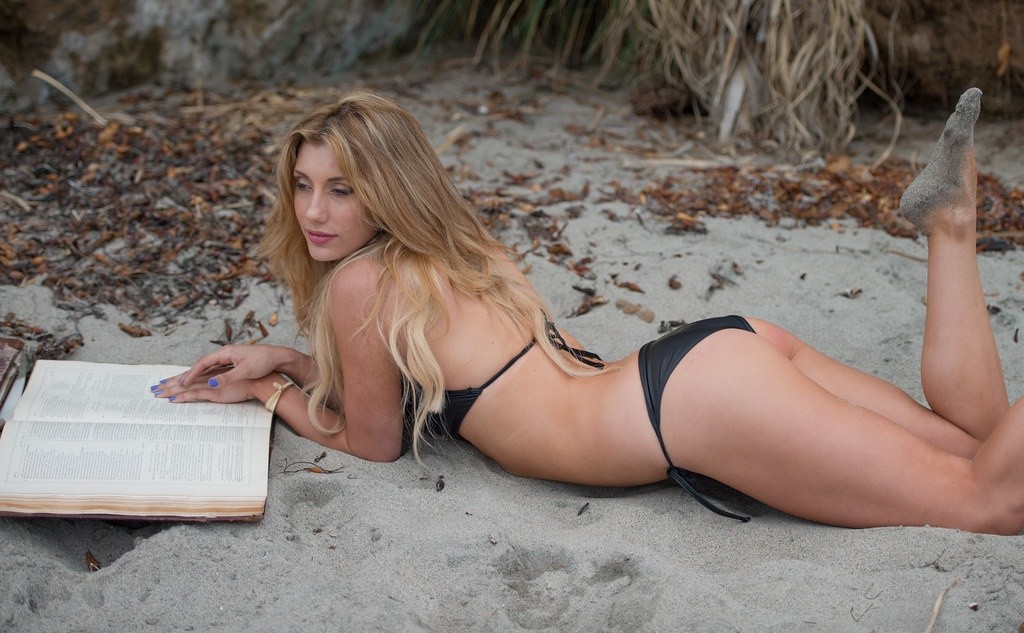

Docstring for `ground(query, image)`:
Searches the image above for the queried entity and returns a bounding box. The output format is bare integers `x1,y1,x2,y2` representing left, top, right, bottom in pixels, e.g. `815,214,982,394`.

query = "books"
0,359,277,522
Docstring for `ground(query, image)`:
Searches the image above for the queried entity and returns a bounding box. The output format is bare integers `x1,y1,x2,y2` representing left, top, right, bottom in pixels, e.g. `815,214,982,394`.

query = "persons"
151,89,1024,538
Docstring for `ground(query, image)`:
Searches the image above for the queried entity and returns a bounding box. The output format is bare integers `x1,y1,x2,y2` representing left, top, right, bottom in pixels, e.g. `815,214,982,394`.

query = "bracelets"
264,381,294,412
